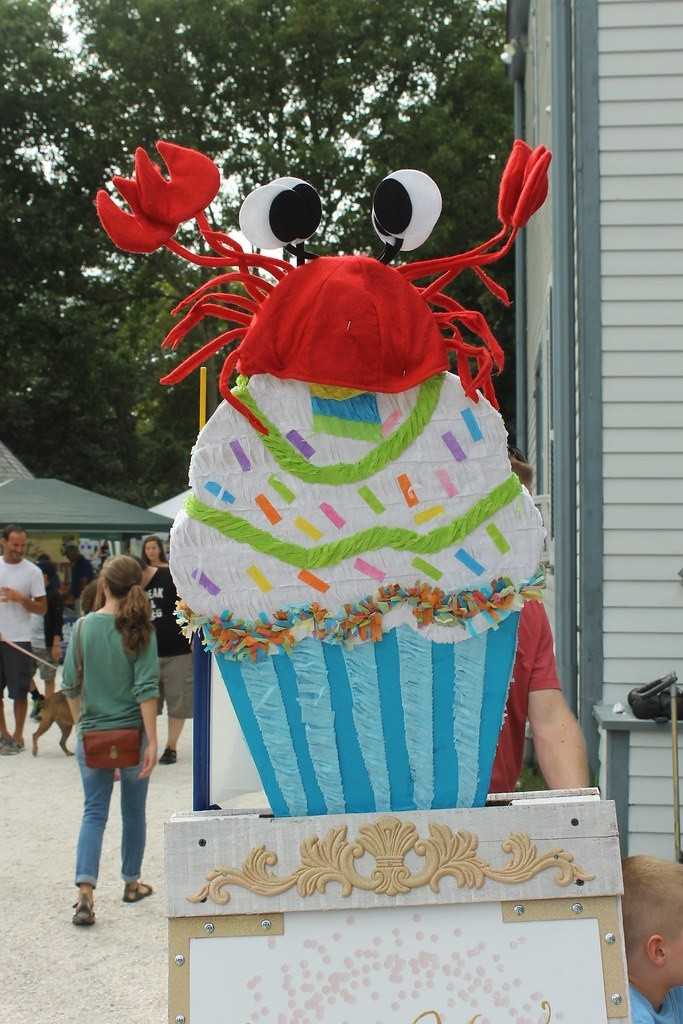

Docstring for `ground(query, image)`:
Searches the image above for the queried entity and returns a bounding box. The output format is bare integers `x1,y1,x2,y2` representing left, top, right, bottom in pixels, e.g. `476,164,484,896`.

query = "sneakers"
29,694,45,723
159,746,177,764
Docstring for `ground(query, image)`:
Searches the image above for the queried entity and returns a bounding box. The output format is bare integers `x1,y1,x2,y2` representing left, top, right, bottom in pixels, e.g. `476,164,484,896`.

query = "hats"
60,542,78,555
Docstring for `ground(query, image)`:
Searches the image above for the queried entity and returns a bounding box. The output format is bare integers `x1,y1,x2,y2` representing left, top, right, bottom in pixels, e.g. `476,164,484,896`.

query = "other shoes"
0,735,24,755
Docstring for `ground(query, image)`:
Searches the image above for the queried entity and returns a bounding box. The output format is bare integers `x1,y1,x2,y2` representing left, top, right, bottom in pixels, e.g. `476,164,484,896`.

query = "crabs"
96,138,553,437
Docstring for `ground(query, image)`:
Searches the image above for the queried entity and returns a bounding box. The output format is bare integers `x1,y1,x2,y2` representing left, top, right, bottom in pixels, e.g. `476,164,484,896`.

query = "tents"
0,477,175,553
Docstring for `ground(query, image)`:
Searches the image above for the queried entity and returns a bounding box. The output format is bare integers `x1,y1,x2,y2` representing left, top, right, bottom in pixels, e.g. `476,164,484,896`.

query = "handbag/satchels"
83,727,140,769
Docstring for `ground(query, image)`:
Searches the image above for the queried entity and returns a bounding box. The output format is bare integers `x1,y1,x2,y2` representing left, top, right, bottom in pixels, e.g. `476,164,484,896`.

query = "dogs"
32,692,75,757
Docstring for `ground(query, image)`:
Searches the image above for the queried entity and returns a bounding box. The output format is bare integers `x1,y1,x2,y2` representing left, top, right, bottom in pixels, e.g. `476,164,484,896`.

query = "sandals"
72,893,95,924
123,882,153,902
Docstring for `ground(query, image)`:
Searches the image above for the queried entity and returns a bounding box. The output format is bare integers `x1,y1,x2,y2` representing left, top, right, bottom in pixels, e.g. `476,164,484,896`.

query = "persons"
621,855,683,1024
487,447,589,805
62,555,161,924
0,525,194,764
